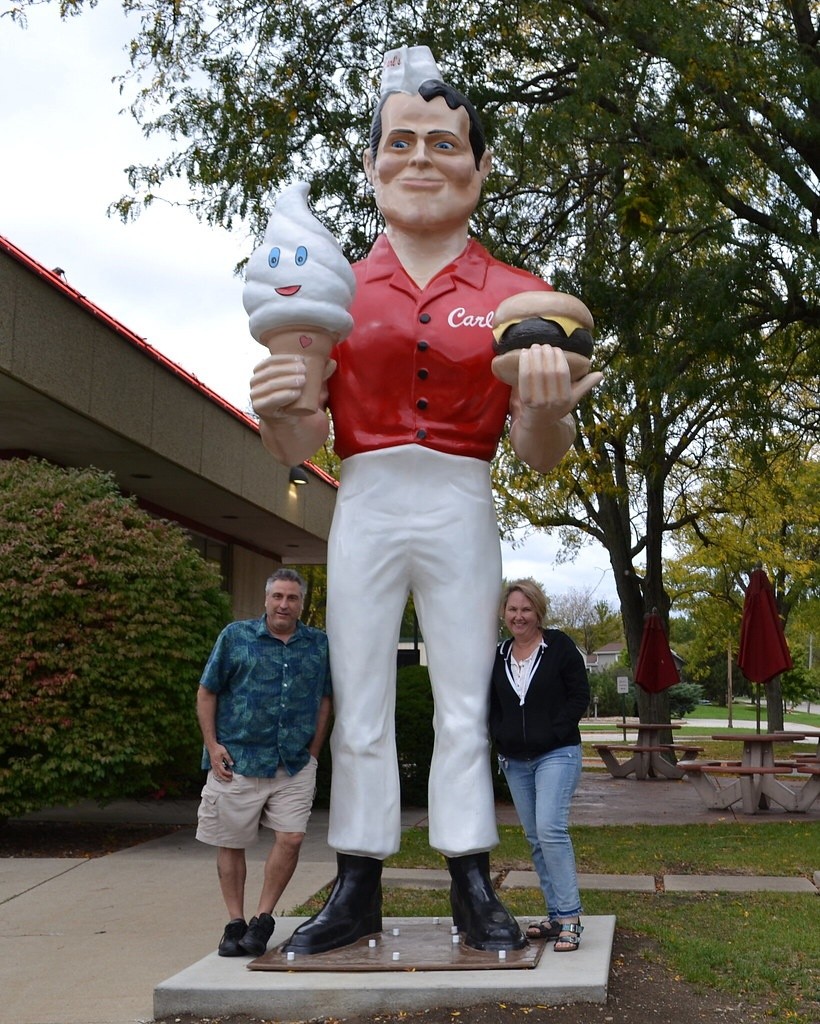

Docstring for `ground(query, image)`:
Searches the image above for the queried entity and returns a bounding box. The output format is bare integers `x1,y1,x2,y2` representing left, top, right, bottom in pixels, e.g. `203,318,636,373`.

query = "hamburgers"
490,290,595,386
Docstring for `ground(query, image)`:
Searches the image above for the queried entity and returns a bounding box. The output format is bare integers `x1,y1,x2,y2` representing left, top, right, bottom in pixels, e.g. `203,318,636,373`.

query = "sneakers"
238,913,275,957
218,918,249,957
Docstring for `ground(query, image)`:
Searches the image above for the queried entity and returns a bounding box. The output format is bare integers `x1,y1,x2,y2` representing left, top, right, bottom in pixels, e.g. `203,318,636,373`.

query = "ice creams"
241,182,358,415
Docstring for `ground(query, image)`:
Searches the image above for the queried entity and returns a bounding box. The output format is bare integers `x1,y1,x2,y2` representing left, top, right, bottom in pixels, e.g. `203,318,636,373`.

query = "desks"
773,730,820,739
589,724,705,780
675,733,820,816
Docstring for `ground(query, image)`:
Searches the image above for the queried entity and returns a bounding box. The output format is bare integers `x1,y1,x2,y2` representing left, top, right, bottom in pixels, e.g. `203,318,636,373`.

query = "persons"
195,568,332,958
250,46,606,950
489,580,590,952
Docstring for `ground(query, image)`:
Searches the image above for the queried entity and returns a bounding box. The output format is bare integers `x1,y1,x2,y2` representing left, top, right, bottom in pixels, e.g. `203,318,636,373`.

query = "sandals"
554,916,584,952
526,918,563,939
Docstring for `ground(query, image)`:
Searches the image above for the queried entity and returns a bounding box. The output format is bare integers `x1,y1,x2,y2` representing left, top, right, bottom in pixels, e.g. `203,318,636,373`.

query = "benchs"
677,752,820,774
591,744,703,753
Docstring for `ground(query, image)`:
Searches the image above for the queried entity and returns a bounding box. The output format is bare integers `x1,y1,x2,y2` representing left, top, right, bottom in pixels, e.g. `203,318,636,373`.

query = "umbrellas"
737,561,793,732
631,607,681,724
